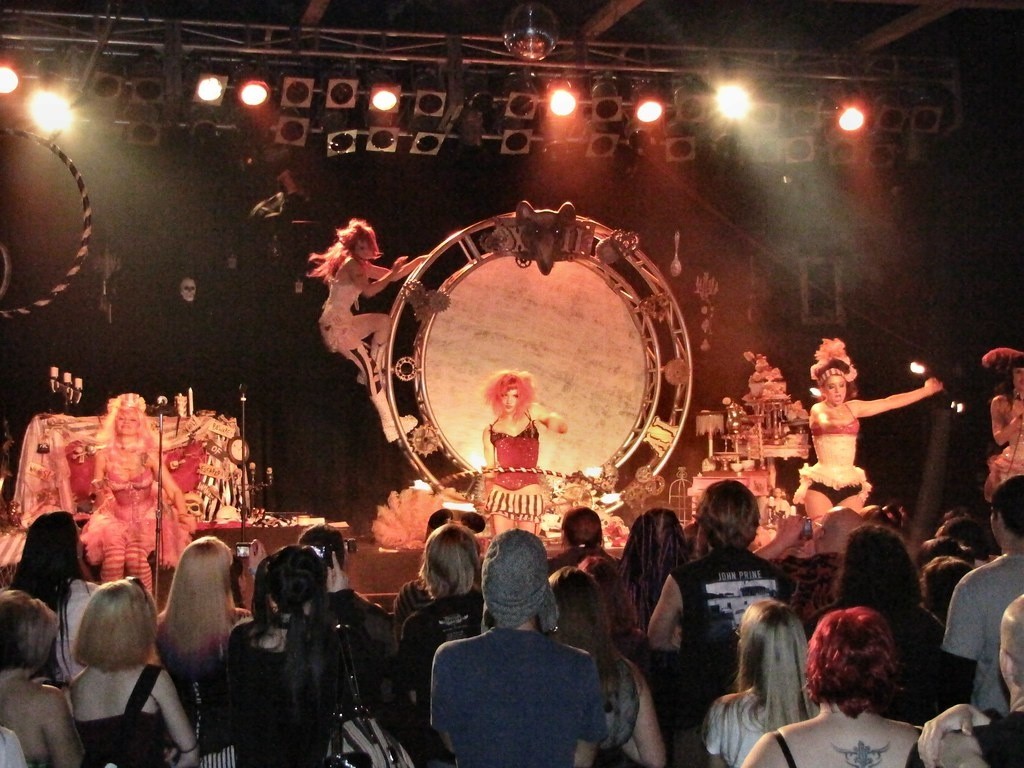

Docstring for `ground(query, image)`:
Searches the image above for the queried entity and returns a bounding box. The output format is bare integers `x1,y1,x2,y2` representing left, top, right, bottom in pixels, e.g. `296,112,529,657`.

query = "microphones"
239,383,247,402
157,396,168,406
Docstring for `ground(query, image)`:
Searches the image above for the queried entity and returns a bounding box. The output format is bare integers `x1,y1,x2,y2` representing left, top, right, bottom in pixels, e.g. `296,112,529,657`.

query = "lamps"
799,257,845,324
0,48,943,171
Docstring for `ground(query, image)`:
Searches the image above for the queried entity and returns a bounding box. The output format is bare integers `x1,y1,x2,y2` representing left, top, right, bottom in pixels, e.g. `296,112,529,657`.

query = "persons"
480,371,568,542
791,336,943,537
617,508,691,627
917,511,991,622
157,534,255,768
298,525,371,706
645,480,797,733
806,525,945,727
546,507,643,658
81,392,195,601
978,348,1024,503
546,566,666,768
704,595,1023,768
224,545,351,767
7,511,100,690
427,527,609,768
306,216,429,444
0,574,197,768
394,509,486,709
942,474,1023,718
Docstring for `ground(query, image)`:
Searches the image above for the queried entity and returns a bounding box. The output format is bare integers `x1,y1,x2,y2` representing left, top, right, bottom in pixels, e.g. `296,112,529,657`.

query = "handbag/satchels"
323,624,413,768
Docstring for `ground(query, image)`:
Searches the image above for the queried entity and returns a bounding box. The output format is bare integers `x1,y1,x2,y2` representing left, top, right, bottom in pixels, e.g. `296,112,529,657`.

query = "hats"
482,530,560,632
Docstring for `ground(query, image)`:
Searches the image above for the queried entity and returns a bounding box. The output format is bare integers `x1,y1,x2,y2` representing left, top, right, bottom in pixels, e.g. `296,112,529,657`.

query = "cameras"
236,542,257,557
798,519,813,542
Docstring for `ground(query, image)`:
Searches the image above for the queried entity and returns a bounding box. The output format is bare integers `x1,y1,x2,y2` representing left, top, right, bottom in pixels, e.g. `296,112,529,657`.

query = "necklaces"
115,441,143,455
825,402,844,415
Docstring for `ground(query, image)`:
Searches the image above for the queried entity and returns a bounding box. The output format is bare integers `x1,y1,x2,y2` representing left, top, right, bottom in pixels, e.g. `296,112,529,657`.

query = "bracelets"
180,743,197,753
